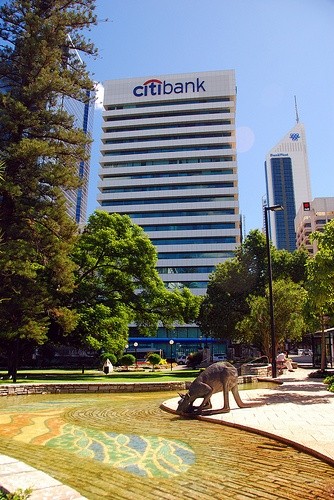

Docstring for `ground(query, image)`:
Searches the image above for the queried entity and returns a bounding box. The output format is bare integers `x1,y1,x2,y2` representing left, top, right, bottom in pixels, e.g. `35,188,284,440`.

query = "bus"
122,337,228,363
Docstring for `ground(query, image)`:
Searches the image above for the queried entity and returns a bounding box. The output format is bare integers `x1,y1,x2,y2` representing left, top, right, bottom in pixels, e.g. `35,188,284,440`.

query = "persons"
190,352,203,370
277,351,295,372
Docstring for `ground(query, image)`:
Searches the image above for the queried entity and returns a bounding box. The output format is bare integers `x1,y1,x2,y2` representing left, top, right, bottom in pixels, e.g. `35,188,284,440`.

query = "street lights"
169,339,174,372
263,203,285,379
134,342,138,360
125,344,129,355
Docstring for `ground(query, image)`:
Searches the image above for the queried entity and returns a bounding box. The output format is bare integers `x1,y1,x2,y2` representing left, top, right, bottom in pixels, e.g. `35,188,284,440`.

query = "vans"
297,349,312,355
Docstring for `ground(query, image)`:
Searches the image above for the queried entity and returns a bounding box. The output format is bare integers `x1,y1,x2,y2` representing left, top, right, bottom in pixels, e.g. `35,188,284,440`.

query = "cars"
211,353,227,363
176,354,186,365
